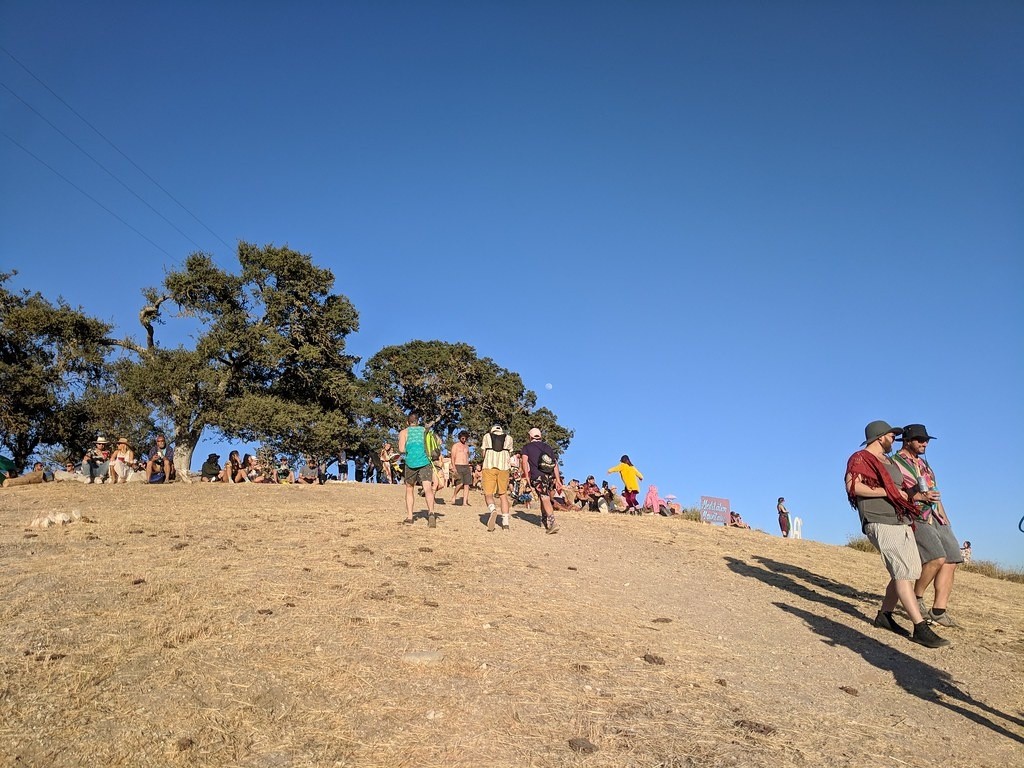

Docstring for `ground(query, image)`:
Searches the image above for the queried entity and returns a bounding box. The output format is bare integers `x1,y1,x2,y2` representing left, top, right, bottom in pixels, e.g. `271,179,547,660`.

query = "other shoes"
540,518,548,529
487,509,497,531
546,522,559,534
404,517,413,526
502,518,509,530
428,511,436,528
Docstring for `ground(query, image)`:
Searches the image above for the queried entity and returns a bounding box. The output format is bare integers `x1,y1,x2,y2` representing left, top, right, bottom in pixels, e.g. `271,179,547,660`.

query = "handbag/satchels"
149,472,165,484
426,432,440,461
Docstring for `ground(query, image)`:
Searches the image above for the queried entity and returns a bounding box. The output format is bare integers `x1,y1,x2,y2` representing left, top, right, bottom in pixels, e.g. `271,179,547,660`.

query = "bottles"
929,481,939,499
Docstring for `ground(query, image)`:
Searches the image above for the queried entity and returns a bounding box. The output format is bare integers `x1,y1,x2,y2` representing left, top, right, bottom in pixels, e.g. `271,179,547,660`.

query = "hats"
859,419,903,447
280,457,287,462
528,427,541,439
117,438,132,447
92,436,109,444
490,426,503,434
895,424,937,441
207,454,220,462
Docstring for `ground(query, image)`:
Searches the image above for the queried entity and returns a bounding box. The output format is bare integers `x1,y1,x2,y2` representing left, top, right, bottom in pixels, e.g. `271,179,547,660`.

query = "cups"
917,476,928,492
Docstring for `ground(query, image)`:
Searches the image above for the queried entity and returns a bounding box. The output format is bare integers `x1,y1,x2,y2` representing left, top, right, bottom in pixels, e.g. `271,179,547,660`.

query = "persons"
844,420,951,648
335,443,405,485
399,412,482,528
564,455,688,516
34,461,44,472
959,541,971,562
65,462,76,473
81,435,175,484
890,424,964,629
201,450,321,485
777,497,791,537
481,424,565,535
5,469,18,478
729,511,751,529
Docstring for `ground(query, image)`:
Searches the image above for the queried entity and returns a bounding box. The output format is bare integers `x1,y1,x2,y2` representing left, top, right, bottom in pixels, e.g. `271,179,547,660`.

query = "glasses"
65,465,73,467
910,437,929,443
883,434,895,443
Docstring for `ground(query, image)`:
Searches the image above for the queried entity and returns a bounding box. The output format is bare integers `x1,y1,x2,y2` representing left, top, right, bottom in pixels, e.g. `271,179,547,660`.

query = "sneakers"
913,621,950,648
928,608,965,632
874,610,910,636
918,598,932,625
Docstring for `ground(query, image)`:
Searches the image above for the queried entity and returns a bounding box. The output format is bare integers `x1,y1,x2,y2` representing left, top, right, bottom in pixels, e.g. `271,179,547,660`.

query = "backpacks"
486,433,509,451
529,442,556,474
